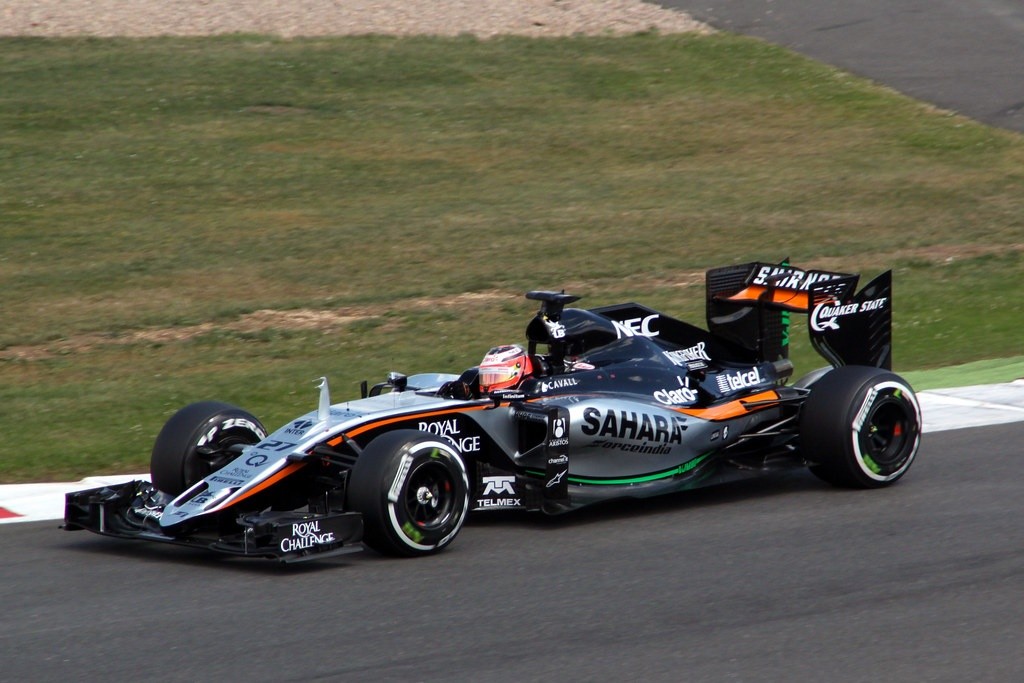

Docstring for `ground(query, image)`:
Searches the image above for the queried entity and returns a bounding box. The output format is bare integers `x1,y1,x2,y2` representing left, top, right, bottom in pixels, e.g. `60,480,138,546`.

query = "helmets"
478,344,534,395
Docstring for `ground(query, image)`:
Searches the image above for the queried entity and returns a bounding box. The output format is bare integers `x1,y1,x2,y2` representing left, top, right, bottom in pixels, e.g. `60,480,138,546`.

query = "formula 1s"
57,258,922,564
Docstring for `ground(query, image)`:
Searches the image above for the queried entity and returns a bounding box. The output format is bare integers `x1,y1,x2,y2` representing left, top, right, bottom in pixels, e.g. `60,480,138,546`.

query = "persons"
476,344,534,398
